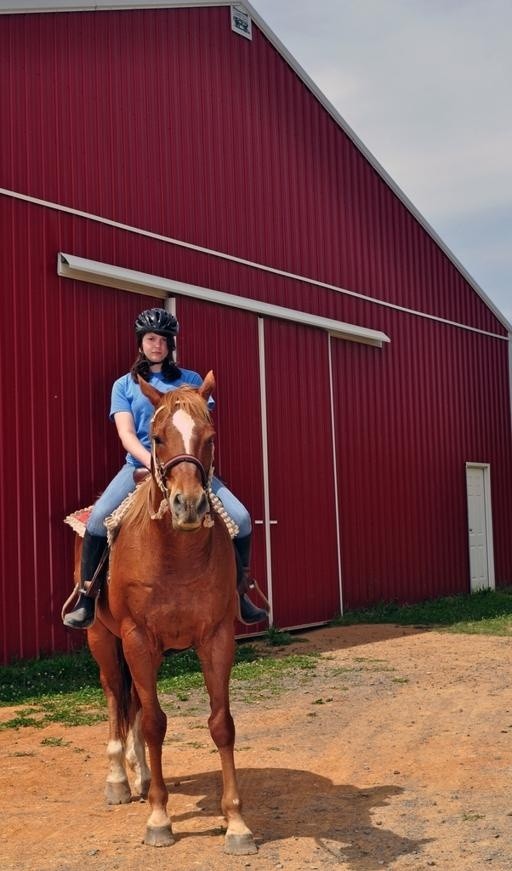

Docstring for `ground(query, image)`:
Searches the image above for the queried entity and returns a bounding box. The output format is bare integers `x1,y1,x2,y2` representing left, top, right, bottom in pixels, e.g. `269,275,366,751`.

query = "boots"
232,529,268,625
63,529,108,628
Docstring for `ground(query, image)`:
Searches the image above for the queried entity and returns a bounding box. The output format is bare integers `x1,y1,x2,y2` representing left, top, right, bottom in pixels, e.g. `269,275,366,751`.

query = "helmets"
135,307,181,337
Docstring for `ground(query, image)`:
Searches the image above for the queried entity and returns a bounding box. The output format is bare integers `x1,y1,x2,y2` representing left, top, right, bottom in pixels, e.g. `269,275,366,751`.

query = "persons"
63,308,269,629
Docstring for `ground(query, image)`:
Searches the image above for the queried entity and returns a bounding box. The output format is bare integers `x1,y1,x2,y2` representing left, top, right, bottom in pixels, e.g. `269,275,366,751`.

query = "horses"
70,367,265,857
